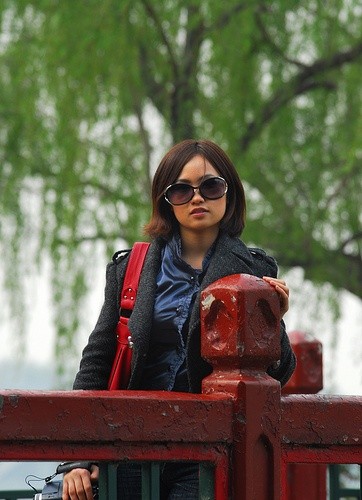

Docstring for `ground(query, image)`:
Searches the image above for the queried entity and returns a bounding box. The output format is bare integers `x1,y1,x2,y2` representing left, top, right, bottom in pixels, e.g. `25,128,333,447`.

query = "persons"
60,140,298,500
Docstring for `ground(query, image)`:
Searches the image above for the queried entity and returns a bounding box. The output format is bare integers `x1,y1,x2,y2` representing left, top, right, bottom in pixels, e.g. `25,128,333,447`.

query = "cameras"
42,479,98,500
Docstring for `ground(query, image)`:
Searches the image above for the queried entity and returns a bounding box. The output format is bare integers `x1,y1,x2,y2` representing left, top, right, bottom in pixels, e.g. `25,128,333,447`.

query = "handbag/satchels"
106,241,149,389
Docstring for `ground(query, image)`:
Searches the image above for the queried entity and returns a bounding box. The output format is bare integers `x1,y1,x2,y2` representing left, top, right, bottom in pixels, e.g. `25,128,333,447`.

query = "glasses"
161,176,228,206
